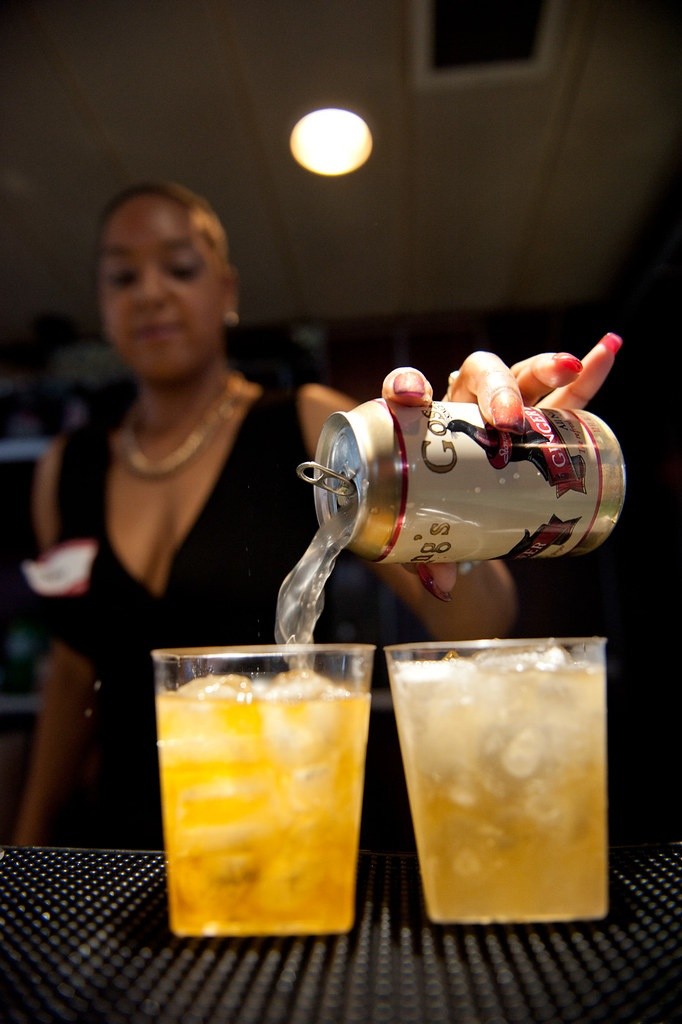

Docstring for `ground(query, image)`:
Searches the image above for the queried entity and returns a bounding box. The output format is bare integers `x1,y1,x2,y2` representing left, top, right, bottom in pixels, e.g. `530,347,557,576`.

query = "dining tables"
1,835,682,1023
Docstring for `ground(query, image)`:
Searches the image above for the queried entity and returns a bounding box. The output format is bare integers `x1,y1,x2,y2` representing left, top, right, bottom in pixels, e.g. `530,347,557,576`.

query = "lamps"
290,107,374,178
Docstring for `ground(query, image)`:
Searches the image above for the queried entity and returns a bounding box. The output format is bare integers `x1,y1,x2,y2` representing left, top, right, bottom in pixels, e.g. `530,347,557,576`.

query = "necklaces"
114,366,244,480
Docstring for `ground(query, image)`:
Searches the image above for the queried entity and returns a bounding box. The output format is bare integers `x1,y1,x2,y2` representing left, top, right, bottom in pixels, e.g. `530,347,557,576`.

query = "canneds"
294,397,627,566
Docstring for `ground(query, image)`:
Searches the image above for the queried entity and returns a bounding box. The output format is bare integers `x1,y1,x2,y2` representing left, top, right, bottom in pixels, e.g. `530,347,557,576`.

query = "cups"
149,637,376,943
383,636,619,926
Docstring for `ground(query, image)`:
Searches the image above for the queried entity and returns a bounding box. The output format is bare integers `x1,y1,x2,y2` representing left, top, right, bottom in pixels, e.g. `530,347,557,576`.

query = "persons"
11,184,621,848
